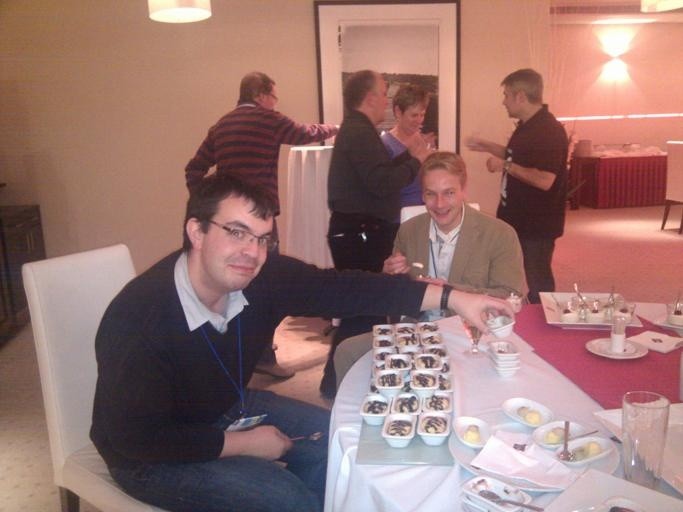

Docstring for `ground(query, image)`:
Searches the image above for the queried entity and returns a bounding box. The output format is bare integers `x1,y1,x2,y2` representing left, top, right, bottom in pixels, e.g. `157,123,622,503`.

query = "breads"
552,428,565,439
464,430,480,445
517,406,529,416
584,443,602,457
467,425,479,432
363,324,451,437
544,431,560,443
524,410,541,425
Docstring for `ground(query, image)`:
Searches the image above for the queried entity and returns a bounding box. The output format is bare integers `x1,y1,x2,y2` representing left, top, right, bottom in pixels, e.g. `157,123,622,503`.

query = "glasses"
207,218,278,252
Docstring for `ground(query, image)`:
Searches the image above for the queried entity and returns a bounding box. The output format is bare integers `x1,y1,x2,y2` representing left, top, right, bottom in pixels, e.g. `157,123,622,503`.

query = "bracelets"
440,283,451,309
503,155,513,174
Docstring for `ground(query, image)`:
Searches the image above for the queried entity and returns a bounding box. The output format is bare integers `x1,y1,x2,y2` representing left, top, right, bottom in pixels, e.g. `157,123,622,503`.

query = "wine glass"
459,312,490,359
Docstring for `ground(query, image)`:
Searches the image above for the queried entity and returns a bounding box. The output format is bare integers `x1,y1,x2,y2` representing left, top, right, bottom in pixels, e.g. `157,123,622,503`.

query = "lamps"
639,1,682,16
148,1,211,25
601,39,627,77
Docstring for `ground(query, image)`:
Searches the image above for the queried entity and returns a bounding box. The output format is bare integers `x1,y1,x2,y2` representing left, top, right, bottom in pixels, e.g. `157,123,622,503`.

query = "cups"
621,391,670,490
560,295,635,324
667,302,683,326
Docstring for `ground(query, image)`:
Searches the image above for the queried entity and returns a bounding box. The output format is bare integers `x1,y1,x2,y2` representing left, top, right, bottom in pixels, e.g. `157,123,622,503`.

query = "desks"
572,156,681,210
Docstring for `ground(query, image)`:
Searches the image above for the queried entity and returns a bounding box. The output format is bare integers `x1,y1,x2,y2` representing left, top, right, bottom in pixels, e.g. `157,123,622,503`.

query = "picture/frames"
314,0,461,157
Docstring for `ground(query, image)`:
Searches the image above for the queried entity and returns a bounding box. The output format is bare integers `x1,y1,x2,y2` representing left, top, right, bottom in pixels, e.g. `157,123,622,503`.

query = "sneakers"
252,363,294,378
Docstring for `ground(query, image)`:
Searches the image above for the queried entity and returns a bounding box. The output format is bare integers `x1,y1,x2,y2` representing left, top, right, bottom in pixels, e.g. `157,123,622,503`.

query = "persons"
463,69,568,306
333,152,530,396
319,69,430,401
90,167,516,512
378,83,436,207
185,71,338,372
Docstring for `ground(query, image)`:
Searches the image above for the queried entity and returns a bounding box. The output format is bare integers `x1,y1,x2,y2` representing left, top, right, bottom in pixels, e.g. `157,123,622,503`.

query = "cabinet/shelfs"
0,203,48,353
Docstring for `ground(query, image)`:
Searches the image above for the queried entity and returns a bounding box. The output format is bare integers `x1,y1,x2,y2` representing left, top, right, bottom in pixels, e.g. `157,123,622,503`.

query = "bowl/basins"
360,320,453,448
486,316,522,377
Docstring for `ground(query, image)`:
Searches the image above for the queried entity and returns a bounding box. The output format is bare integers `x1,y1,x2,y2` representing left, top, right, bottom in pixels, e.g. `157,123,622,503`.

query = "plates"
449,397,683,512
586,338,649,360
538,292,644,330
634,300,683,336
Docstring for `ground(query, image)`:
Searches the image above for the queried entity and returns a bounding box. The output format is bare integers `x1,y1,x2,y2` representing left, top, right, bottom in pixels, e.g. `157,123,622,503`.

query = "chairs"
21,244,165,512
661,141,683,236
400,203,481,222
